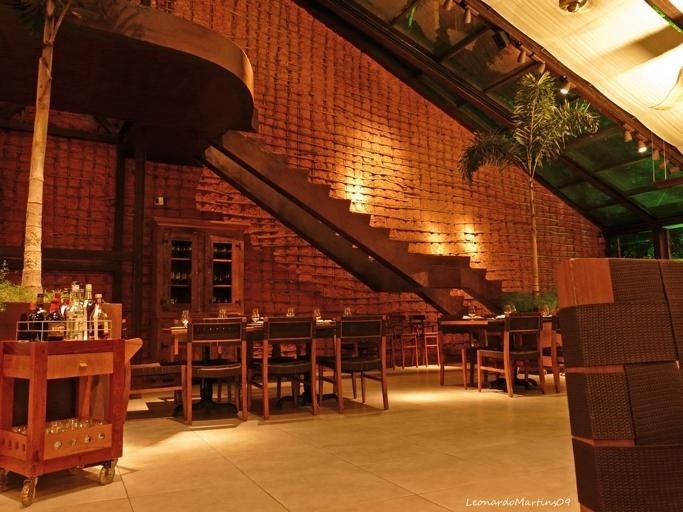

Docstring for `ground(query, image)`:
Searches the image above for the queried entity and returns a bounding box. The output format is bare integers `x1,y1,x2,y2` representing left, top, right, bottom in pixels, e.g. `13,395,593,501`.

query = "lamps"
444,0,680,174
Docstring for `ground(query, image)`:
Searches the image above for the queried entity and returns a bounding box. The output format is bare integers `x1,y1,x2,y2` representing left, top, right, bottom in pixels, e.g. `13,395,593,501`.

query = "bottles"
18,283,110,341
172,243,230,261
213,290,230,302
171,265,230,285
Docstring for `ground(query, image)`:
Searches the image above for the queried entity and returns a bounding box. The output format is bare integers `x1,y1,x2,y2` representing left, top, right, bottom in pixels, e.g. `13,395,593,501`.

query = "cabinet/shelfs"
166,235,236,361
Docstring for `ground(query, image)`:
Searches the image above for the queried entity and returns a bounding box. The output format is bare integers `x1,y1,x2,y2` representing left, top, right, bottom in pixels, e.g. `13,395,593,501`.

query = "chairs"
180,314,388,425
391,313,563,397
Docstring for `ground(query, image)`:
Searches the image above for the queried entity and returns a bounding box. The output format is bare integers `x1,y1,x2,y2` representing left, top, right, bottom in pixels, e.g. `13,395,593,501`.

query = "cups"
216,309,226,318
285,307,295,316
502,304,516,316
15,419,105,434
341,306,352,317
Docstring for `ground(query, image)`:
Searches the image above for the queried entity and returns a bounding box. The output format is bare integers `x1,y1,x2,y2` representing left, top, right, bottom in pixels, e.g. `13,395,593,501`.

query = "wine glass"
251,308,259,324
311,305,320,321
467,306,476,322
181,310,190,328
